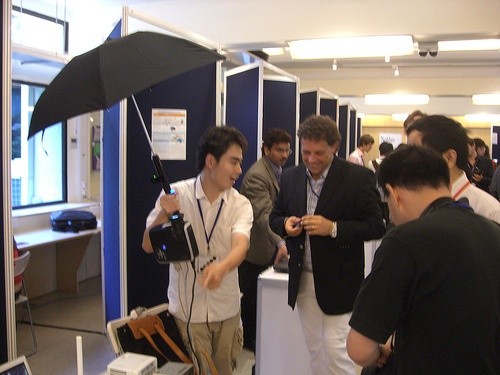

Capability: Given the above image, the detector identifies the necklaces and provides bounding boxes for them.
[453,182,470,199]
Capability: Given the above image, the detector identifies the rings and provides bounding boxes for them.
[312,224,315,229]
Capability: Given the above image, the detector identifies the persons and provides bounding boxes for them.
[369,142,393,224]
[268,115,386,375]
[466,137,500,203]
[403,111,426,127]
[142,126,254,375]
[13,236,22,300]
[346,146,500,375]
[240,127,292,375]
[349,134,375,166]
[407,115,500,224]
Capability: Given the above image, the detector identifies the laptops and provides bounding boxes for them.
[0,355,33,375]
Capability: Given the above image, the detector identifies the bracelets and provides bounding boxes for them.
[330,222,335,235]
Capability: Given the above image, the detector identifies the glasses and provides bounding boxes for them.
[272,148,293,155]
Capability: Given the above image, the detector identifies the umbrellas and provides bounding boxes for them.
[28,31,226,193]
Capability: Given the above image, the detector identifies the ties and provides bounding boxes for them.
[361,156,365,167]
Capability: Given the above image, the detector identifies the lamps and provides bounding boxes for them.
[266,32,500,121]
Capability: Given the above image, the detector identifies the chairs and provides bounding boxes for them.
[14,250,37,360]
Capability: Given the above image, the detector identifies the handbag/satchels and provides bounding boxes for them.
[50,210,97,233]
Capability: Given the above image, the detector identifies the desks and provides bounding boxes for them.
[15,219,100,297]
[255,268,310,375]
